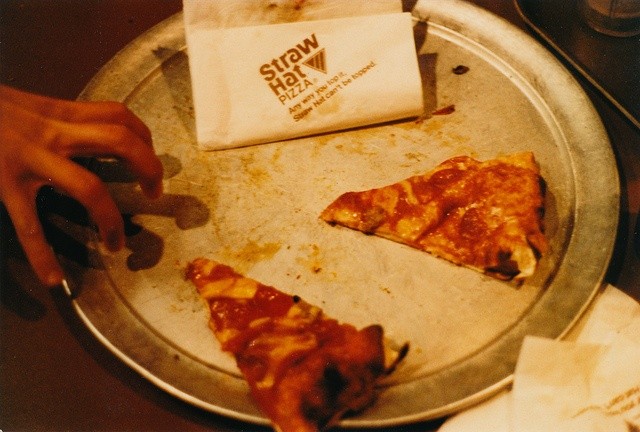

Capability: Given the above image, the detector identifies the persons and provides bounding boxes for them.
[1,84,163,288]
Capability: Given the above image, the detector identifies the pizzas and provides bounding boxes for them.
[320,149,543,288]
[188,256,409,432]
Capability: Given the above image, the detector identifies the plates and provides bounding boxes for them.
[57,3,625,428]
